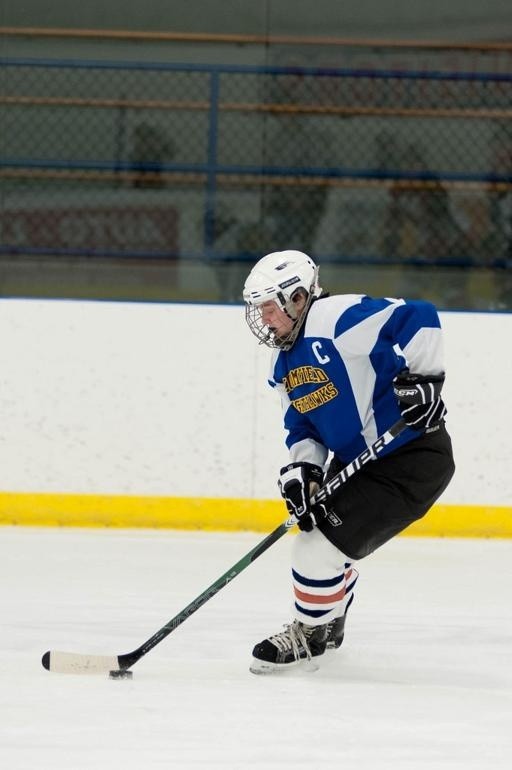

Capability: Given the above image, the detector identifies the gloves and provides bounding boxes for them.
[393,369,453,436]
[272,458,332,530]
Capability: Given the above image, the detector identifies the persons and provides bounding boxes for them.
[242,250,456,664]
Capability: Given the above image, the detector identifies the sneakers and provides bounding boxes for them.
[332,607,350,649]
[251,618,332,666]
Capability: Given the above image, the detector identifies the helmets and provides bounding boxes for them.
[236,248,321,354]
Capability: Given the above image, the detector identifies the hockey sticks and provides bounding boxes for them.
[42,421,409,675]
